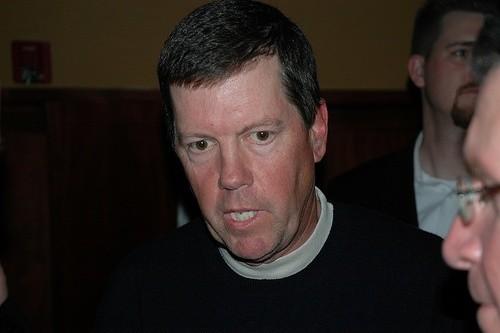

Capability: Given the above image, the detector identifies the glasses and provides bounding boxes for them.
[455,176,496,223]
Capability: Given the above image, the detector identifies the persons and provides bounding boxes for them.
[325,0,500,240]
[94,0,478,333]
[440,13,500,333]
